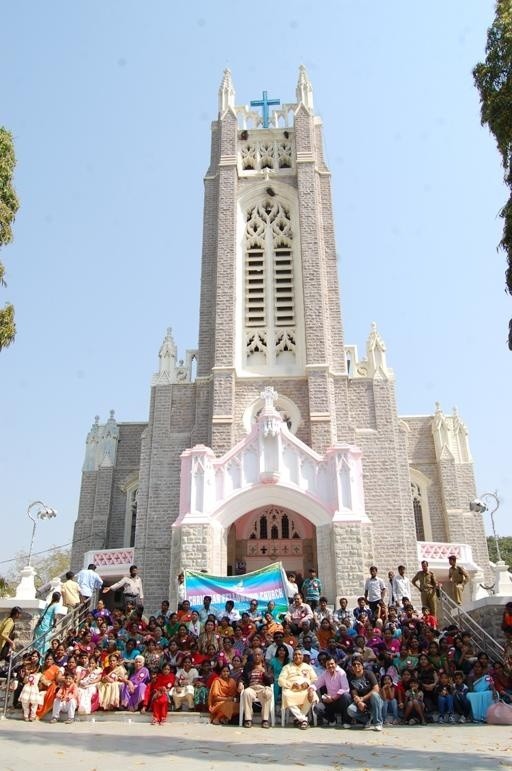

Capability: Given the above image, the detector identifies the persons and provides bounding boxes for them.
[364,567,386,608]
[447,556,469,605]
[177,574,185,605]
[1,607,22,679]
[283,569,300,603]
[103,565,144,608]
[33,592,62,655]
[301,568,322,609]
[412,561,436,615]
[18,595,512,733]
[62,572,84,610]
[73,564,104,603]
[392,566,410,607]
[387,571,397,606]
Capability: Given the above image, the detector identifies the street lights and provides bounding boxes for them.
[16,501,57,600]
[468,490,512,594]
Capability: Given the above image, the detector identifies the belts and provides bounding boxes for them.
[125,593,137,598]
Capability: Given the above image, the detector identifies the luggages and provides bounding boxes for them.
[467,691,498,721]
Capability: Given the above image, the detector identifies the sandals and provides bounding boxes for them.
[211,717,229,725]
[299,720,310,730]
[292,719,301,729]
[261,720,269,729]
[244,719,252,728]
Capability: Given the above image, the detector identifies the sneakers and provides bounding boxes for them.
[363,715,383,732]
[141,709,166,725]
[329,719,336,727]
[342,721,352,729]
[437,714,472,724]
[385,719,426,726]
[25,716,74,725]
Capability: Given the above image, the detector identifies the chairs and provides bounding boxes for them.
[239,684,275,726]
[281,687,317,727]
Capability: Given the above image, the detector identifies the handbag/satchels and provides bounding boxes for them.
[473,675,493,691]
[435,588,440,599]
[487,701,512,725]
